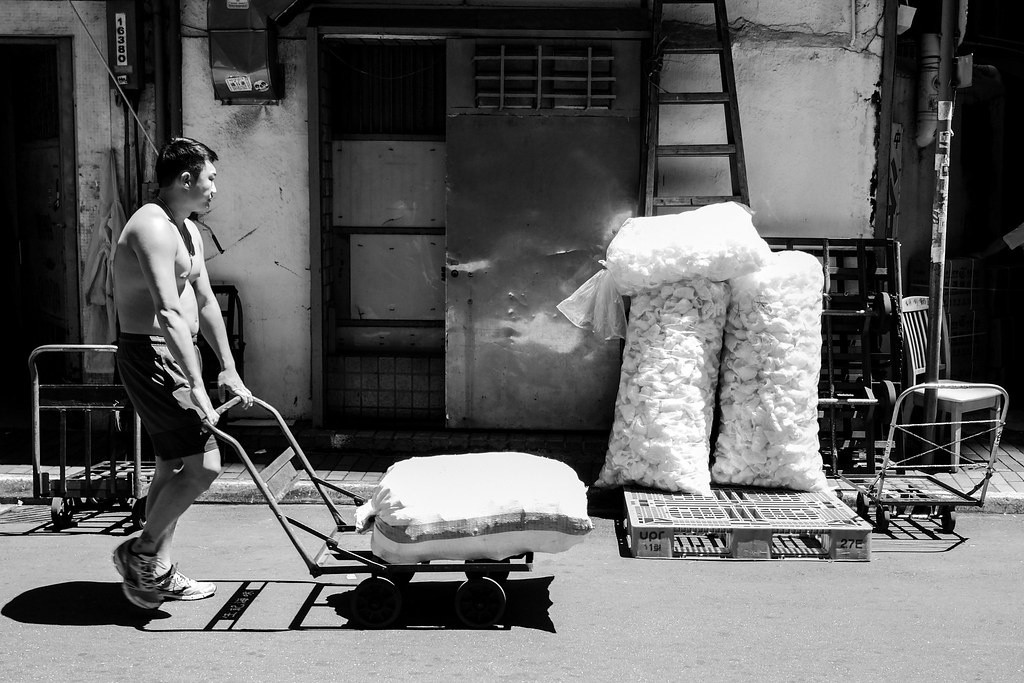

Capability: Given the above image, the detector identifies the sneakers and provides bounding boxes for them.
[155,562,218,603]
[111,536,167,610]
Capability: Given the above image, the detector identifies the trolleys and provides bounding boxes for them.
[839,381,1009,533]
[28,344,159,530]
[201,393,535,626]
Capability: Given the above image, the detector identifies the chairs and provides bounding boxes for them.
[899,294,1004,472]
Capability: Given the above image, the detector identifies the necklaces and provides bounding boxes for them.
[158,196,196,256]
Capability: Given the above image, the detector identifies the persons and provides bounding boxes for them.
[113,137,253,609]
